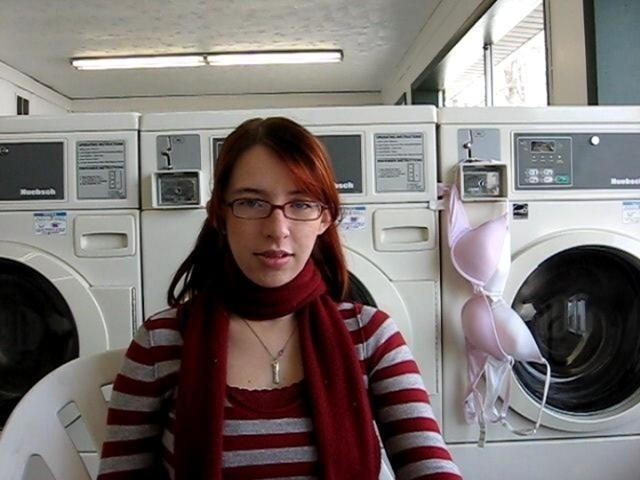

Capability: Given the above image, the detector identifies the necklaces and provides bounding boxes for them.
[235,314,300,385]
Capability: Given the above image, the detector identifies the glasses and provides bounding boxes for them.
[223,198,328,221]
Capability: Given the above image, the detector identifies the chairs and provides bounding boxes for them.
[1,351,129,479]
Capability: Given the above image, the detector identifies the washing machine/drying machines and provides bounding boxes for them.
[142,105,442,480]
[0,112,141,479]
[438,105,640,480]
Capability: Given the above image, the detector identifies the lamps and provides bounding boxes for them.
[69,50,346,72]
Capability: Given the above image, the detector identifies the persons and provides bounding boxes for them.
[95,118,463,480]
